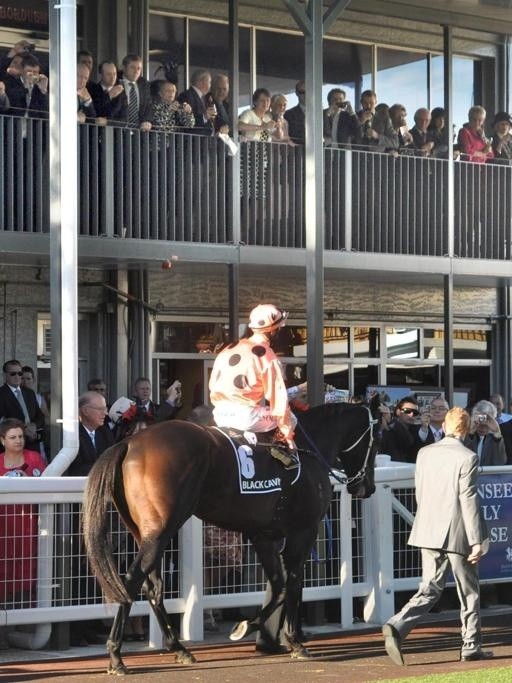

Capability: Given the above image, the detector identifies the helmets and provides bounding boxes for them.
[247,303,287,333]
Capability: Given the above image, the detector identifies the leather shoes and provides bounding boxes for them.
[460,652,494,662]
[382,623,405,667]
[122,633,145,642]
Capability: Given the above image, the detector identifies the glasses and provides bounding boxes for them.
[93,388,104,392]
[6,372,23,377]
[400,406,419,417]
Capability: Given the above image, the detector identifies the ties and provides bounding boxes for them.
[126,83,138,128]
[14,389,30,425]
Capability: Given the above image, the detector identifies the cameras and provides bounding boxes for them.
[476,415,487,423]
[336,101,347,109]
[176,387,181,393]
[31,74,39,82]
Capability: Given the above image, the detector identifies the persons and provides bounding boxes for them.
[1,38,512,259]
[204,292,300,466]
[381,406,490,669]
[4,356,507,640]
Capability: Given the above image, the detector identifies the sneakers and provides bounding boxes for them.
[70,623,110,646]
[271,440,298,468]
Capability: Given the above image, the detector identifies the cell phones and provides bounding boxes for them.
[426,404,431,418]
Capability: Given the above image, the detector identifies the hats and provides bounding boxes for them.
[491,112,512,126]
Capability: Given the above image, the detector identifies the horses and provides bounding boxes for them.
[79,393,383,676]
